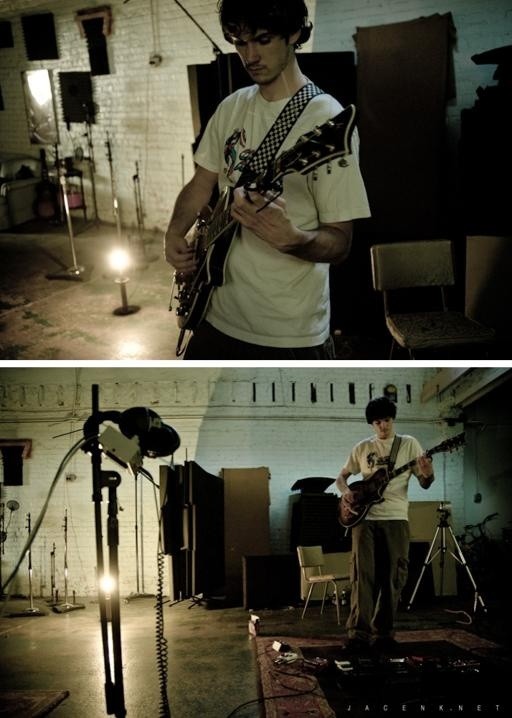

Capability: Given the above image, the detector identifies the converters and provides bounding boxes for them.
[272,640,291,652]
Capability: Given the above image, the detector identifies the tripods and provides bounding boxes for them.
[407,516,488,613]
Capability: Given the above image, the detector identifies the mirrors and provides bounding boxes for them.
[21,69,59,144]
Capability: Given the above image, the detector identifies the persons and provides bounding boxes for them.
[334,395,434,654]
[161,0,371,361]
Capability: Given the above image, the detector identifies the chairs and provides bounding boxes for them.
[296,545,350,625]
[370,239,495,360]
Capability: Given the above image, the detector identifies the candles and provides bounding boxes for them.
[106,248,132,281]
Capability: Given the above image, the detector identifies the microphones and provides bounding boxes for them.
[168,453,176,471]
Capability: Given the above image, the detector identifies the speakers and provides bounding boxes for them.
[242,554,300,610]
[289,493,352,555]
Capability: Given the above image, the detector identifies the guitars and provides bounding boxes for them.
[175,103,358,329]
[32,148,59,219]
[337,433,464,528]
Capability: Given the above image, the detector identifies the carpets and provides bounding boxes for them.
[252,626,512,718]
[0,690,69,718]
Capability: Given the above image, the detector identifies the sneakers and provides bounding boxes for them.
[342,636,392,648]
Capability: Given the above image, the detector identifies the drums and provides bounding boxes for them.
[66,192,82,208]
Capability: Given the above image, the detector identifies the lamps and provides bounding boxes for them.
[149,54,162,67]
